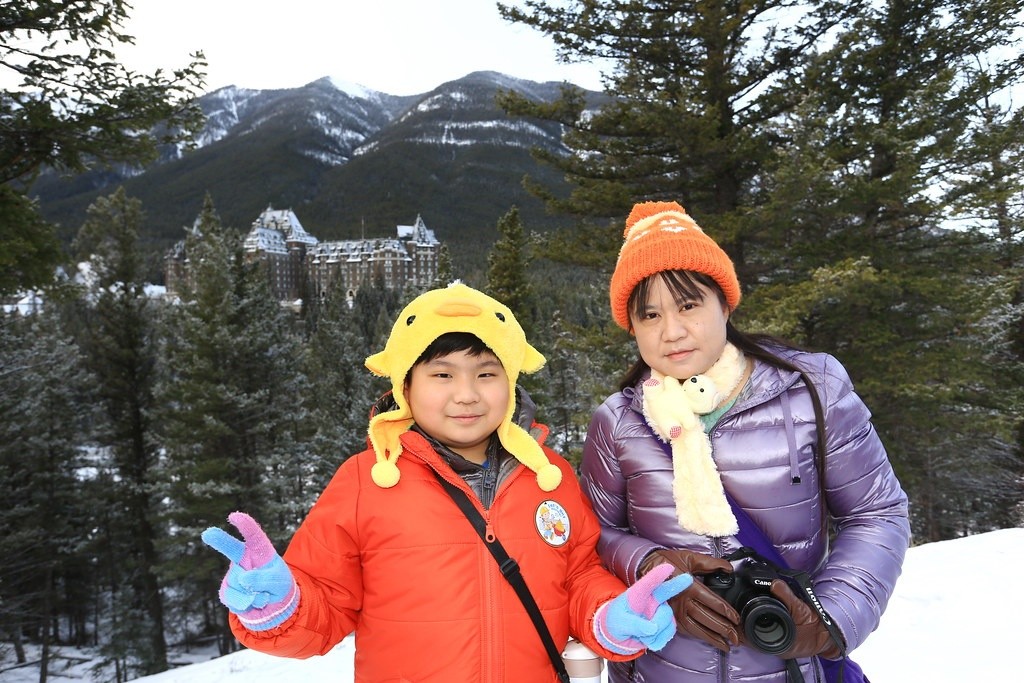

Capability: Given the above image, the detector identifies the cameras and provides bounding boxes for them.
[701,560,797,657]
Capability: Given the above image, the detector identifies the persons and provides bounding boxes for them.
[578,202,910,683]
[201,280,693,683]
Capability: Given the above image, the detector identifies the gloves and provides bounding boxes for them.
[639,548,740,654]
[735,579,844,661]
[200,511,299,632]
[592,562,693,656]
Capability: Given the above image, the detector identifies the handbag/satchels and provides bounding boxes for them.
[822,656,870,683]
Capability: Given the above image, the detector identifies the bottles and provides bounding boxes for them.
[561,640,604,683]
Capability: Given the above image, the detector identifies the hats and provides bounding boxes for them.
[365,280,562,492]
[610,201,741,336]
